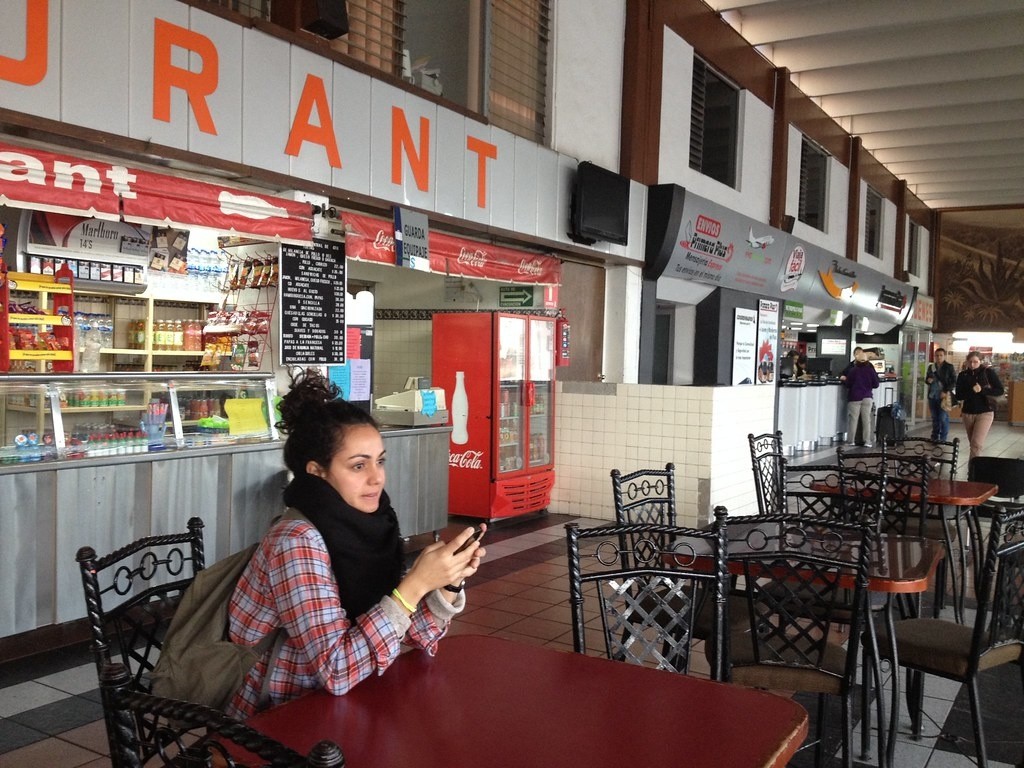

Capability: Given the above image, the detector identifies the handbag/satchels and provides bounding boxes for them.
[981,366,1002,412]
[940,387,963,413]
[144,505,332,732]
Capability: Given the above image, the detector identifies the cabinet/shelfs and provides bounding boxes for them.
[0,263,73,374]
[0,290,236,445]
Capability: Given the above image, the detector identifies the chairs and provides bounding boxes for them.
[75,515,347,768]
[563,429,1024,768]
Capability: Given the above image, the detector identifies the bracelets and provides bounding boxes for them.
[393,589,417,612]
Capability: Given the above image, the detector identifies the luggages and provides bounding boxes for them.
[873,387,905,447]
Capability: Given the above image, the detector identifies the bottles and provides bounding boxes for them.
[55,310,215,372]
[497,380,548,472]
[450,371,470,445]
[167,394,222,420]
[8,390,149,463]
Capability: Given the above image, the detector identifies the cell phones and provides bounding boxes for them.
[454,527,482,554]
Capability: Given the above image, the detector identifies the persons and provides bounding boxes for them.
[925,348,956,441]
[787,350,808,377]
[224,368,486,722]
[840,347,879,448]
[956,351,1004,457]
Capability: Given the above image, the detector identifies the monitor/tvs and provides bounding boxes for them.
[571,160,630,248]
[779,357,794,380]
[806,358,831,380]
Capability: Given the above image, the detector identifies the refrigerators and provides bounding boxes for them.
[429,312,557,526]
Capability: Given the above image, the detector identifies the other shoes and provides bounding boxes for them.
[863,443,873,448]
[844,442,856,446]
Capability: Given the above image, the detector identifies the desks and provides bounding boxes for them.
[809,474,999,626]
[658,520,945,768]
[208,635,809,768]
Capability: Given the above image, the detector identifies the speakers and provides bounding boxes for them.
[302,0,348,41]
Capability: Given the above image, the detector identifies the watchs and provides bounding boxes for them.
[444,579,465,593]
